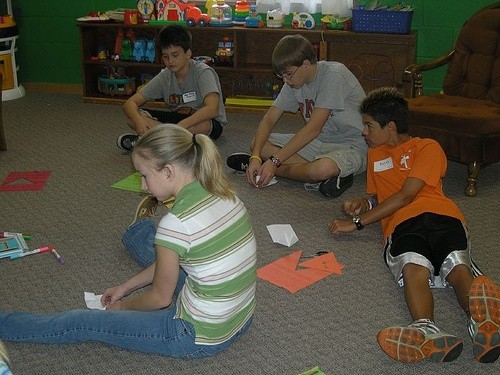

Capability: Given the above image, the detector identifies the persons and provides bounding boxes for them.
[327,87,500,363]
[0,123,257,358]
[116,24,228,151]
[226,34,370,197]
[0,341,13,375]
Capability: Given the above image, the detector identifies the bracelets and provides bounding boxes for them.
[249,155,262,164]
[363,198,376,212]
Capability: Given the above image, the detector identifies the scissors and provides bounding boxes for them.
[301,251,329,257]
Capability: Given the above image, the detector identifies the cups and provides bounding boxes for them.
[124,10,137,25]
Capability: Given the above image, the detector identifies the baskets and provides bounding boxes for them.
[352,9,414,34]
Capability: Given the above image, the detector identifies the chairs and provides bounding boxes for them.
[402,2,500,195]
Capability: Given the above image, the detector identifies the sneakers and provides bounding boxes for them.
[227,152,251,174]
[376,318,463,365]
[467,275,500,363]
[117,132,138,151]
[128,195,159,227]
[319,172,354,198]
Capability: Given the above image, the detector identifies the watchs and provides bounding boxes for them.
[269,155,282,167]
[352,215,364,230]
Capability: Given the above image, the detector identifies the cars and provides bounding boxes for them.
[186,7,210,27]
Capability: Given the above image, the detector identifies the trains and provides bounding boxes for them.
[214,35,233,66]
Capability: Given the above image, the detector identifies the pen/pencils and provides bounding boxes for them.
[0,232,32,240]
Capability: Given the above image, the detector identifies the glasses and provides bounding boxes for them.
[275,66,300,80]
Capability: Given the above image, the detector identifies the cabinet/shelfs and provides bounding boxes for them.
[77,20,418,116]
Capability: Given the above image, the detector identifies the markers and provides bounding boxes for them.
[10,247,49,259]
[50,246,65,264]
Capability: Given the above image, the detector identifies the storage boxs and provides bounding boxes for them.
[98,76,135,94]
